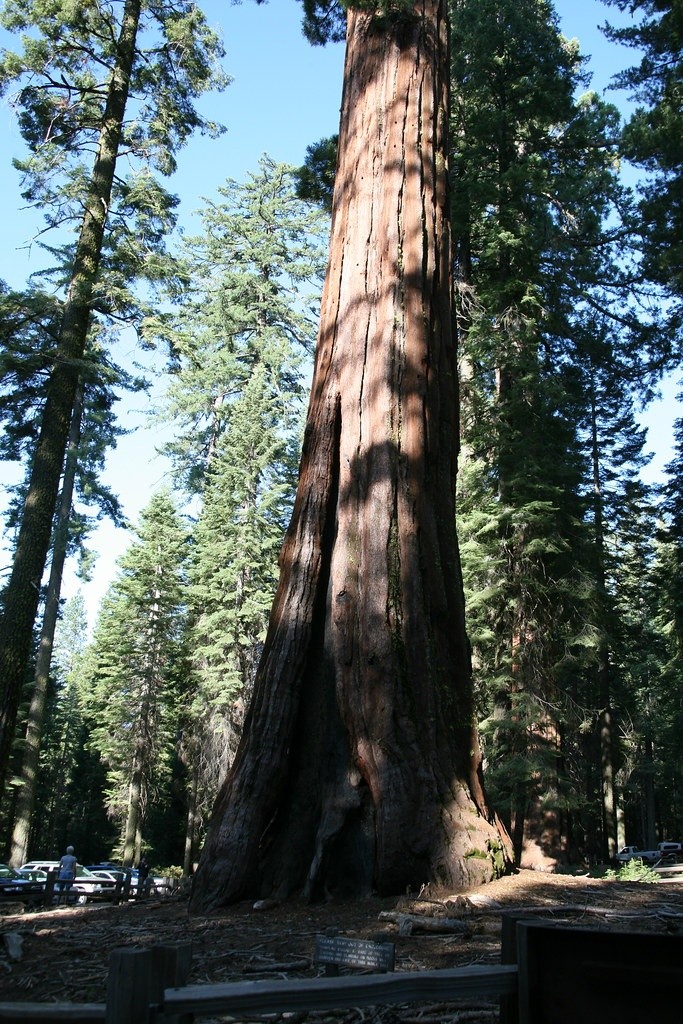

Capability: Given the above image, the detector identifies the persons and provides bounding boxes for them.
[135,857,151,881]
[57,846,76,905]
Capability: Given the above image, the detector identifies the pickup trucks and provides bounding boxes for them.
[615,846,661,867]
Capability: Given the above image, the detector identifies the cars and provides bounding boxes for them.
[85,865,153,883]
[657,842,683,863]
[20,861,117,904]
[0,868,79,906]
[0,863,45,906]
[82,870,157,899]
